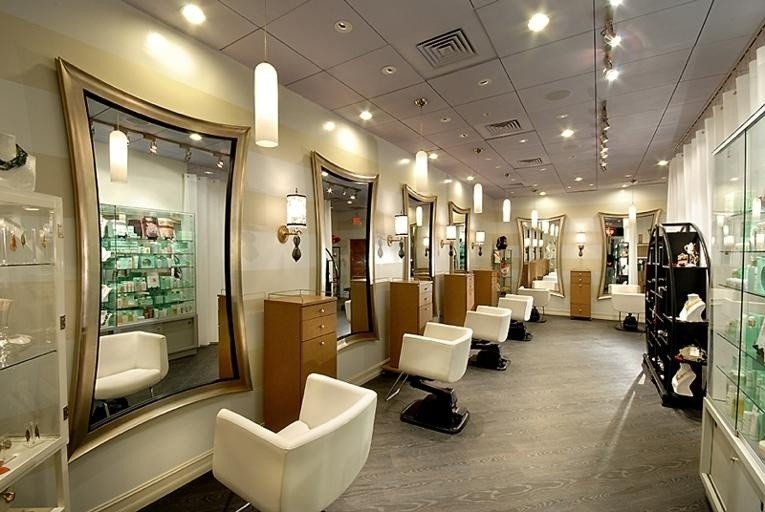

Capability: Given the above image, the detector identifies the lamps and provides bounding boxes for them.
[524,239,543,248]
[629,204,636,221]
[599,0,620,175]
[276,188,486,262]
[538,221,559,237]
[575,231,586,257]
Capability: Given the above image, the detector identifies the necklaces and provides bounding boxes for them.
[676,370,688,380]
[685,298,702,311]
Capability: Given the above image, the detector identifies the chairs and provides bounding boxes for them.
[612,293,646,332]
[532,269,557,290]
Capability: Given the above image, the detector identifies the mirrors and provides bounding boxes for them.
[516,213,565,299]
[597,209,662,300]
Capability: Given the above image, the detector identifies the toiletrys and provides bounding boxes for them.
[723,191,765,462]
[103,239,193,327]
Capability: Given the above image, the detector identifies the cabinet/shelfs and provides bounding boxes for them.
[264,269,497,432]
[699,104,765,511]
[570,270,592,320]
[0,189,71,512]
[493,249,512,298]
[642,221,710,411]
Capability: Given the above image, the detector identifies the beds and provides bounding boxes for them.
[608,284,640,294]
